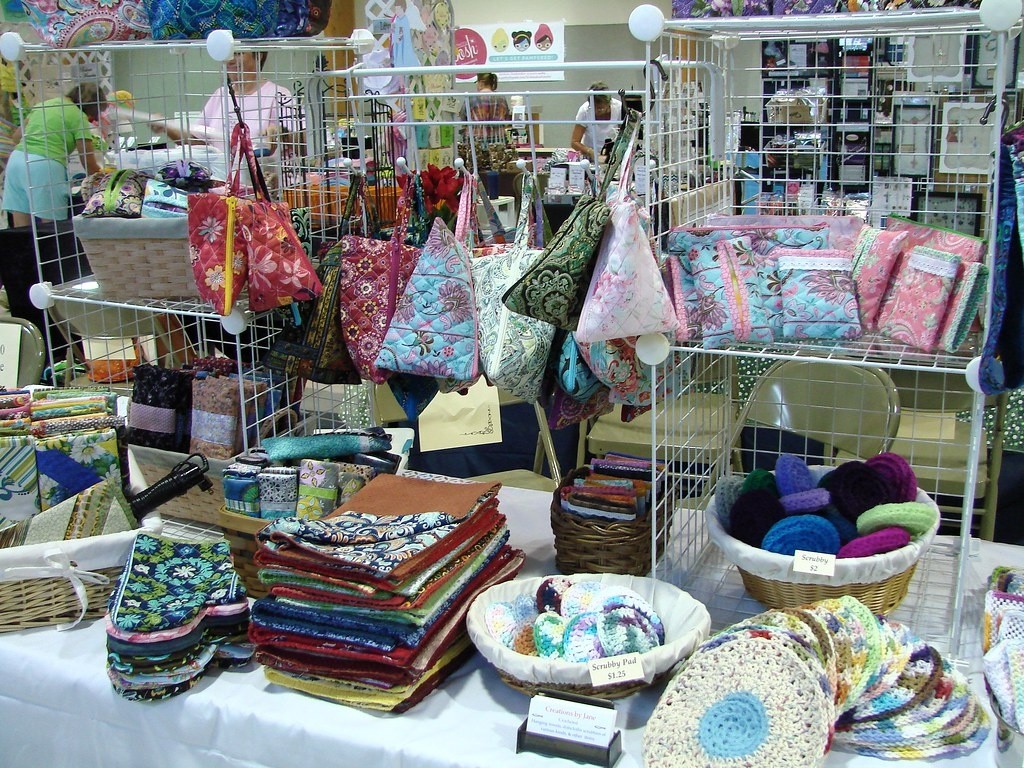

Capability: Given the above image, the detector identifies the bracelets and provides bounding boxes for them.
[587,148,593,155]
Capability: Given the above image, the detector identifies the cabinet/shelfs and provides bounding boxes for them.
[631,1,1022,662]
[0,30,375,457]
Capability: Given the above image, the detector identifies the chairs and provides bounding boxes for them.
[27,280,182,386]
[577,355,746,496]
[375,375,562,493]
[703,354,901,496]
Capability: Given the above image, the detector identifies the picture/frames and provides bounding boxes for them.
[971,32,1020,89]
[511,106,544,148]
[870,169,916,219]
[938,102,994,174]
[891,104,934,177]
[907,29,967,82]
[911,191,983,237]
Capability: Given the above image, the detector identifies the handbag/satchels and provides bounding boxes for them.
[82,159,217,217]
[186,107,680,428]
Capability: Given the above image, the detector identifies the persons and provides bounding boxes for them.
[571,82,621,160]
[2,82,108,227]
[146,51,306,195]
[461,74,510,150]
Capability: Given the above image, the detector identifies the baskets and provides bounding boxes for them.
[551,465,670,578]
[72,197,198,298]
[466,573,711,699]
[0,448,162,634]
[705,465,941,622]
[127,394,317,528]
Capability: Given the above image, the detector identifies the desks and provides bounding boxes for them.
[0,443,1024,768]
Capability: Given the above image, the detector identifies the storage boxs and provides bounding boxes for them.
[130,442,249,526]
[765,98,828,123]
